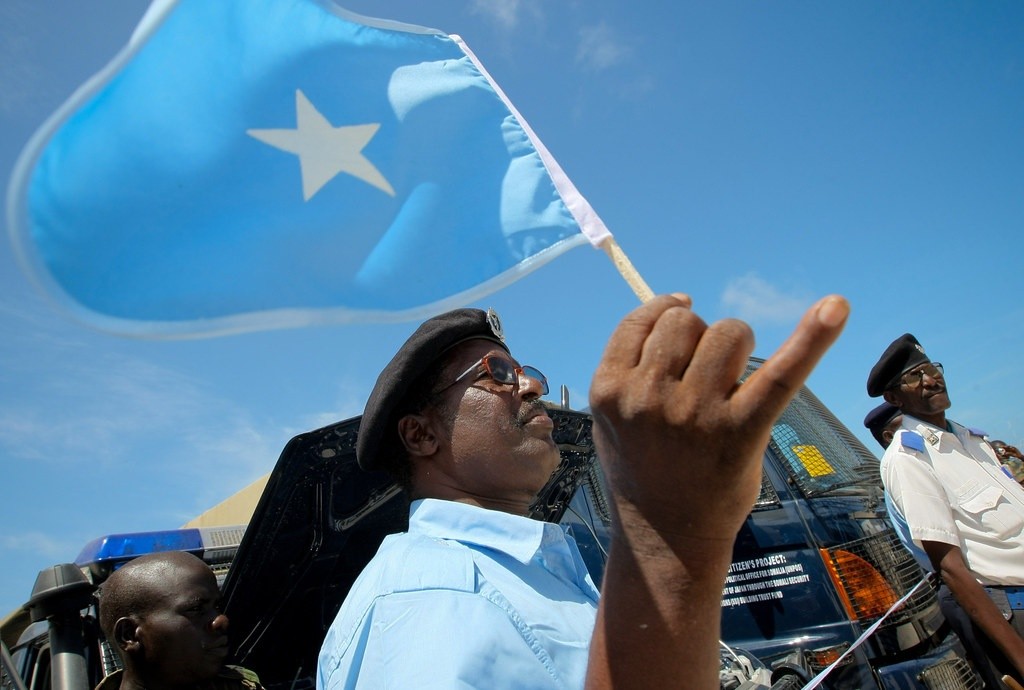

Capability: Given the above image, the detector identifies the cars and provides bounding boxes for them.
[0,398,594,689]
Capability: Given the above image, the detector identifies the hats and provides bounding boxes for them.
[355,306,511,471]
[864,401,902,432]
[867,333,930,397]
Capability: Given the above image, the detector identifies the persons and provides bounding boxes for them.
[865,334,1024,690]
[94,551,266,690]
[315,292,849,690]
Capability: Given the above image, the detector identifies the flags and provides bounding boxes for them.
[6,1,613,339]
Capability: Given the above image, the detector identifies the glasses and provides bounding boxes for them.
[884,362,944,391]
[420,350,550,409]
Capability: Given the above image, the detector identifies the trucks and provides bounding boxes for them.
[559,356,986,690]
